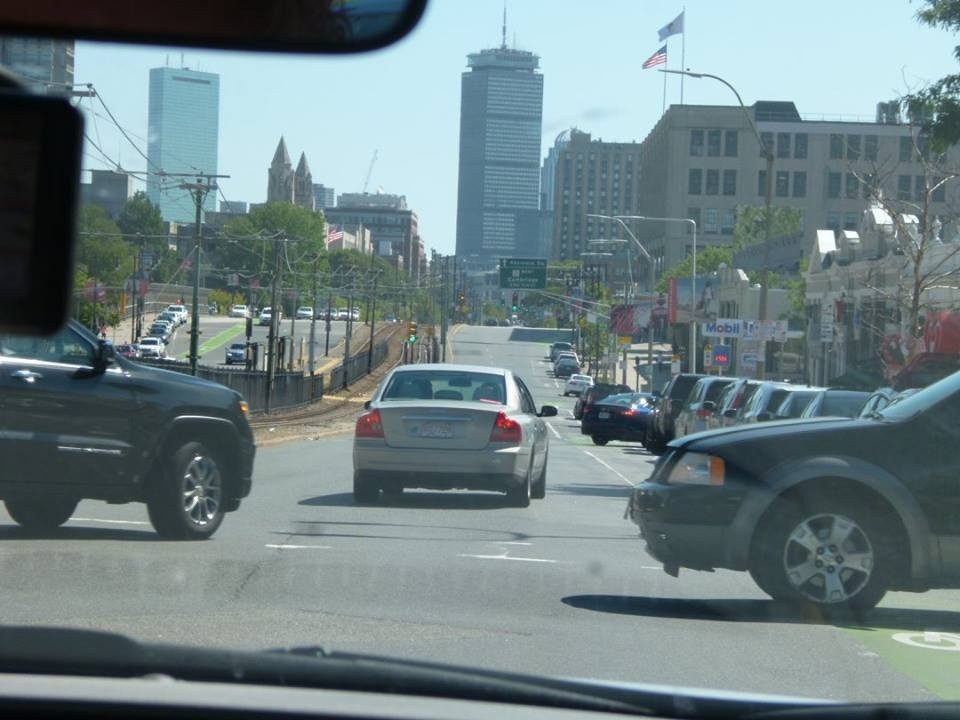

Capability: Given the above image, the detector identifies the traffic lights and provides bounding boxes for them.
[460,293,464,304]
[407,322,417,343]
[512,292,518,313]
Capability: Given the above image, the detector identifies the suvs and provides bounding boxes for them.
[644,369,960,621]
[0,315,256,542]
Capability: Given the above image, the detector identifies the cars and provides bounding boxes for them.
[296,306,359,321]
[565,374,594,396]
[231,305,248,317]
[485,318,510,327]
[225,343,249,364]
[573,383,657,446]
[353,365,558,507]
[115,304,187,357]
[549,341,580,377]
[259,307,282,324]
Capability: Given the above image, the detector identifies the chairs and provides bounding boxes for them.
[434,389,463,400]
[473,382,502,401]
[399,383,423,397]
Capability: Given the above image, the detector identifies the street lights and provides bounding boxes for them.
[612,214,696,374]
[658,67,774,380]
[585,214,657,395]
